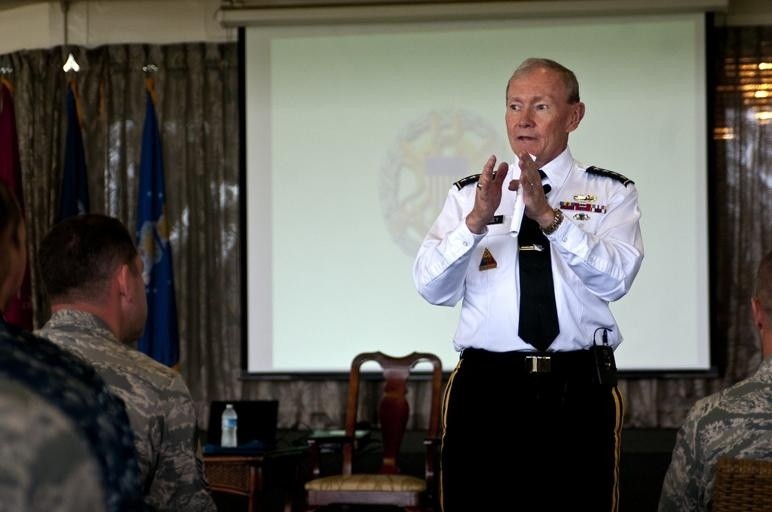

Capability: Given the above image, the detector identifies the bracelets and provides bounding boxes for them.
[544,208,563,235]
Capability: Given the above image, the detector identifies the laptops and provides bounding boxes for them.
[201,400,279,457]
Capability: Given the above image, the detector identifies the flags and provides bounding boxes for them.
[2,77,178,370]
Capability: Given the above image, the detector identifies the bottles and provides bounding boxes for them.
[221,404,238,449]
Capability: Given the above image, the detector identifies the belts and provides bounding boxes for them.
[462,355,594,374]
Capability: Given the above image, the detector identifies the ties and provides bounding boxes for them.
[518,169,560,352]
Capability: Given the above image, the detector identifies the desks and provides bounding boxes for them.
[201,428,428,512]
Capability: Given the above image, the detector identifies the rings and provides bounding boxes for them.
[477,183,482,187]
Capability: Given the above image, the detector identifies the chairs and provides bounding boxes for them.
[712,456,772,512]
[304,352,441,512]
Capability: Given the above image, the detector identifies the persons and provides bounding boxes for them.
[412,59,644,512]
[659,252,772,512]
[1,178,216,512]
